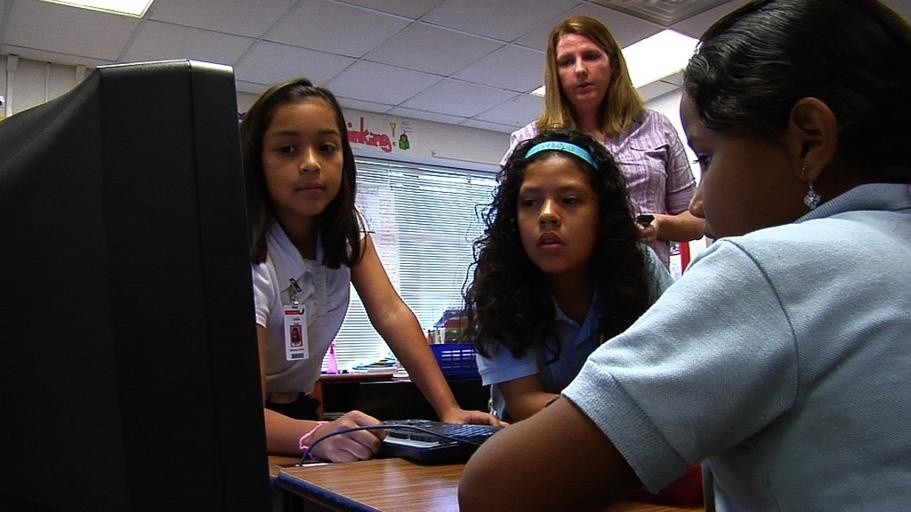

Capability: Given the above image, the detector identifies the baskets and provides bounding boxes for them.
[428,344,481,380]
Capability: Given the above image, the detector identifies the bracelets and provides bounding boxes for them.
[298,419,330,459]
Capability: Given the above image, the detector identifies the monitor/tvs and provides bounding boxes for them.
[1,58,279,512]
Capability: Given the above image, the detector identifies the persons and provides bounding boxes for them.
[291,327,302,347]
[457,0,911,511]
[463,126,678,423]
[493,16,707,273]
[239,75,511,463]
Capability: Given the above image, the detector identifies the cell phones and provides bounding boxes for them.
[637,215,655,227]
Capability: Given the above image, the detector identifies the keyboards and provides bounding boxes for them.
[379,418,504,465]
